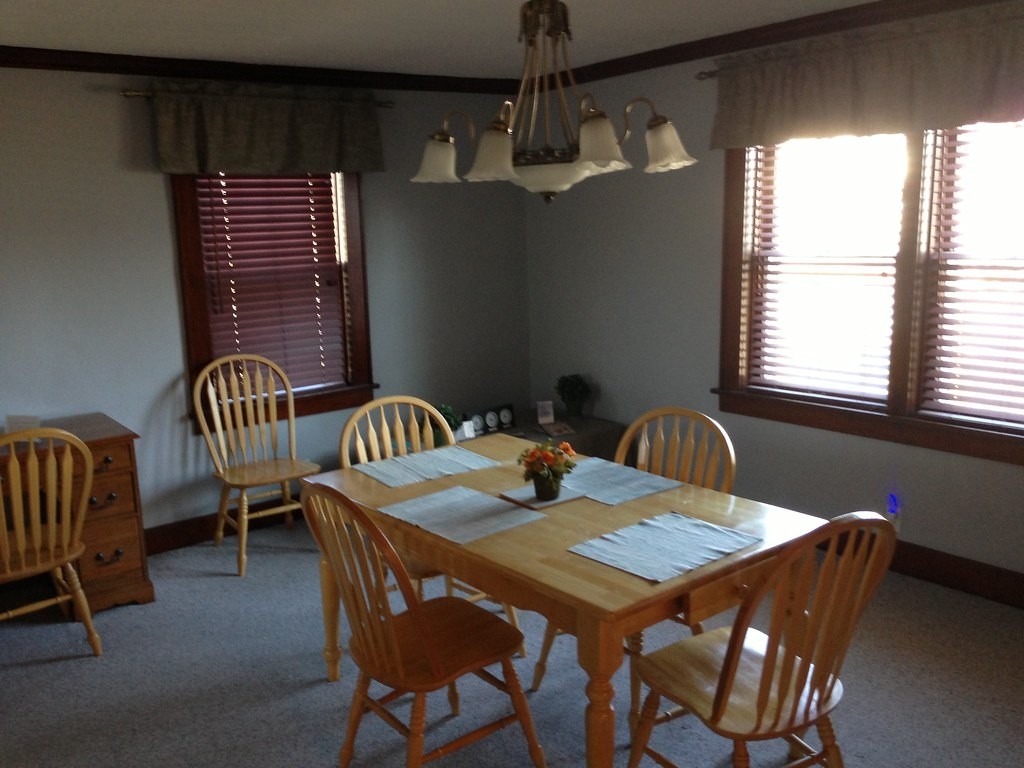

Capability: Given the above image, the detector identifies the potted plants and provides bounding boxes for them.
[554,372,589,418]
[416,403,463,449]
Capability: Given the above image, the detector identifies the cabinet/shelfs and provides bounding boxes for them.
[0,409,159,616]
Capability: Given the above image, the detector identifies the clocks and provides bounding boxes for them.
[467,403,517,433]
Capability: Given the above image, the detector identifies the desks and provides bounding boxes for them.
[381,413,627,462]
[301,433,830,768]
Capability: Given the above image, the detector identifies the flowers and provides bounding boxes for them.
[517,438,576,488]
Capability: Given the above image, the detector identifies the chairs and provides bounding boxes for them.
[301,483,548,768]
[0,427,105,657]
[337,396,525,659]
[531,408,736,747]
[193,353,324,576]
[621,512,896,768]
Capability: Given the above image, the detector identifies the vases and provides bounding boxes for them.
[534,472,561,499]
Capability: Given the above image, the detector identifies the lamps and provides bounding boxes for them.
[409,0,697,203]
